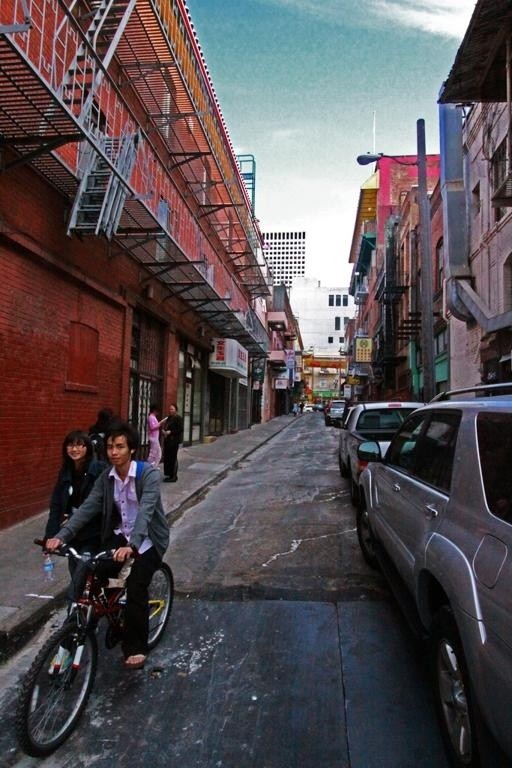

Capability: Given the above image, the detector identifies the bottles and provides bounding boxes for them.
[42,550,55,583]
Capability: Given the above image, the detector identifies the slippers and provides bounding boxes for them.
[125,652,149,669]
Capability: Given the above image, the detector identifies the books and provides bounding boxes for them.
[158,416,169,424]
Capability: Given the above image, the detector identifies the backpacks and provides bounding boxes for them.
[88,433,106,460]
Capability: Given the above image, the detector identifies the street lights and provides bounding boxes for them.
[356,149,437,401]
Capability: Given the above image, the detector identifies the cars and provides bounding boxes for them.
[303,399,347,427]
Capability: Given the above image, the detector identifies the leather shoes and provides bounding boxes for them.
[163,476,177,482]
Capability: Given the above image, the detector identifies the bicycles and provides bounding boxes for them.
[18,537,173,757]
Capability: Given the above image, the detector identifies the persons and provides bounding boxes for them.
[145,402,169,471]
[43,418,171,672]
[161,402,184,483]
[292,401,298,416]
[300,402,304,415]
[41,428,110,686]
[87,406,117,466]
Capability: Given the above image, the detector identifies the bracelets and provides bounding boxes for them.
[126,542,139,558]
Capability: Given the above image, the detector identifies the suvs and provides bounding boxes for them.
[355,379,511,766]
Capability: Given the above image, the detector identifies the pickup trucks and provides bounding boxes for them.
[339,401,431,508]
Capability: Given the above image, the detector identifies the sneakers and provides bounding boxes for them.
[48,654,73,675]
